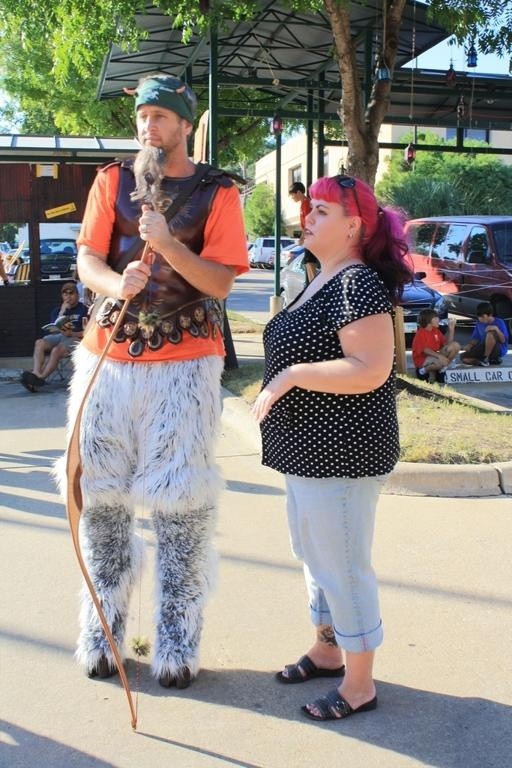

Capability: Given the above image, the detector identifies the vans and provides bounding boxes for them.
[401,212,512,324]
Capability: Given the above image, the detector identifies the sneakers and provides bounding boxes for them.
[436,370,446,383]
[478,355,489,367]
[490,356,502,364]
[415,367,429,381]
[20,371,46,392]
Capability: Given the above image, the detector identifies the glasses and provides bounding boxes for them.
[334,173,363,218]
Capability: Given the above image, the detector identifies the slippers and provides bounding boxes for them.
[300,687,377,721]
[276,653,346,683]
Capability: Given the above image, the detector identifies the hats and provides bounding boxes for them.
[61,283,78,293]
[123,74,198,122]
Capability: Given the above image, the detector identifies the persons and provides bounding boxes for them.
[287,181,319,285]
[249,171,416,726]
[0,251,14,290]
[457,299,509,368]
[48,72,255,694]
[18,281,91,396]
[410,308,462,385]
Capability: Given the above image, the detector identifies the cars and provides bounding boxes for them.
[387,269,453,350]
[245,236,308,270]
[0,233,78,280]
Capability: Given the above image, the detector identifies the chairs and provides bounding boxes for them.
[48,308,84,383]
